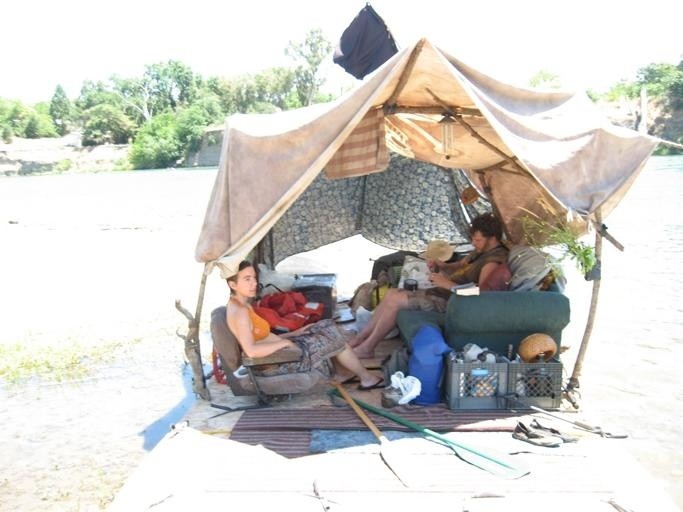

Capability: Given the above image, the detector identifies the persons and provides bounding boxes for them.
[346,212,510,360]
[227,259,386,391]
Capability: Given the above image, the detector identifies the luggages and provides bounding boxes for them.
[371,284,391,308]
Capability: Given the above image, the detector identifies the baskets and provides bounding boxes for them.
[444,352,507,411]
[508,356,562,409]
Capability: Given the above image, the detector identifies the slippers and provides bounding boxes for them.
[359,376,384,390]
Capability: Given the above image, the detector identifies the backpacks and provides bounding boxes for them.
[348,280,376,317]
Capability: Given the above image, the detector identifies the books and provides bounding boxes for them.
[449,281,480,297]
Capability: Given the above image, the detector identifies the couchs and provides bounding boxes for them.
[387,246,570,359]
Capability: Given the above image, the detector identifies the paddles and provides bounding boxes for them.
[324,375,443,489]
[328,384,531,479]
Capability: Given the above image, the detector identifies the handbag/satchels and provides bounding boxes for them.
[381,342,409,384]
[403,325,451,407]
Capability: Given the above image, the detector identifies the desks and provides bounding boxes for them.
[292,272,338,320]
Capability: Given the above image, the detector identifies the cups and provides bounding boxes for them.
[403,278,418,291]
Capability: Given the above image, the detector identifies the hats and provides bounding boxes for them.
[422,240,454,263]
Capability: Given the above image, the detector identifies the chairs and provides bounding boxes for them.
[210,306,319,406]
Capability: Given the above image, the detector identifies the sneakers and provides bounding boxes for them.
[529,419,577,442]
[381,386,403,407]
[512,422,564,446]
